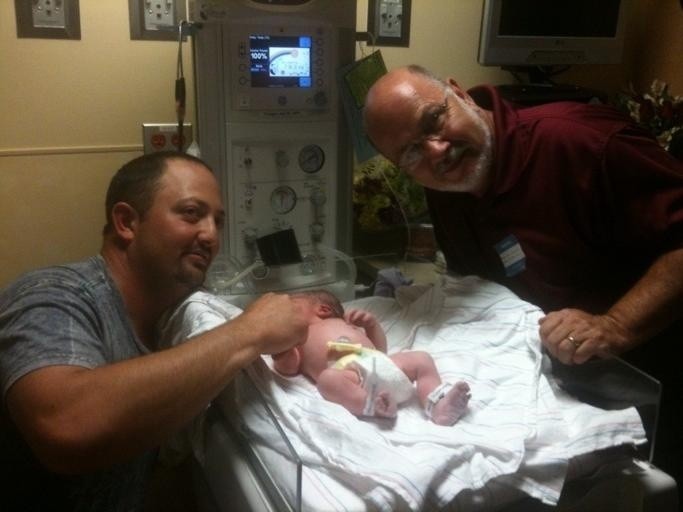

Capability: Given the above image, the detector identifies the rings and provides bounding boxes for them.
[566,336,579,348]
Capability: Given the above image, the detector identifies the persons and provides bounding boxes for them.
[270,288,473,427]
[360,63,683,511]
[0,151,312,512]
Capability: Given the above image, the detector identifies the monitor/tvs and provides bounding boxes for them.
[476,0,623,66]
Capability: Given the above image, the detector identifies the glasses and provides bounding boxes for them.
[396,89,456,183]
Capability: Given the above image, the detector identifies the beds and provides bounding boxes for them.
[180,282,664,511]
